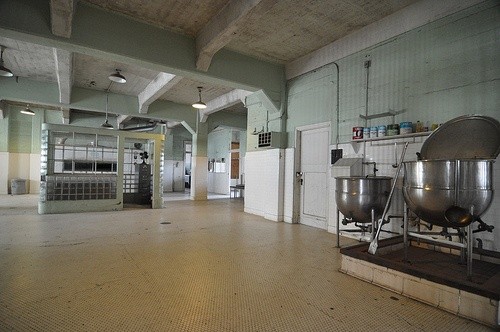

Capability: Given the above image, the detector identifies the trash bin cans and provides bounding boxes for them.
[11,179,27,195]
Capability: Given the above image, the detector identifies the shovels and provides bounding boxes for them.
[368,141,410,255]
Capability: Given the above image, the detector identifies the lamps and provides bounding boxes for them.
[191,87,207,108]
[0,45,14,77]
[109,69,127,83]
[19,103,36,115]
[100,93,113,127]
